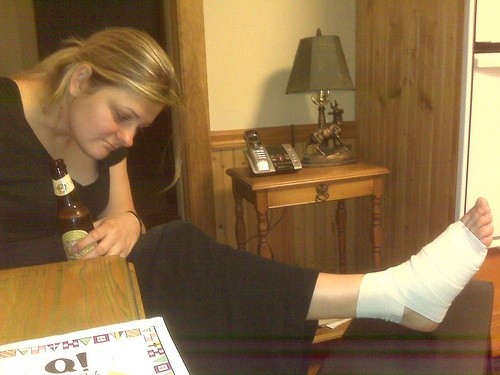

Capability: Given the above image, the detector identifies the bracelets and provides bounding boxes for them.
[126,210,143,237]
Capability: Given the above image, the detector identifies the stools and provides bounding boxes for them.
[315,279,494,375]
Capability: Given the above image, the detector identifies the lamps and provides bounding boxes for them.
[286,28,358,167]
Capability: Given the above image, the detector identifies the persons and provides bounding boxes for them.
[0,27,495,375]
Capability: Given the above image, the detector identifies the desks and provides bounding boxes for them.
[225,161,391,273]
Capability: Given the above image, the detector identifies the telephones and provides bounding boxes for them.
[243,124,302,177]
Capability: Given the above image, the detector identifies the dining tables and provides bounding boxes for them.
[0,255,147,345]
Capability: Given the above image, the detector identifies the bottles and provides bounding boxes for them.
[49,158,104,261]
